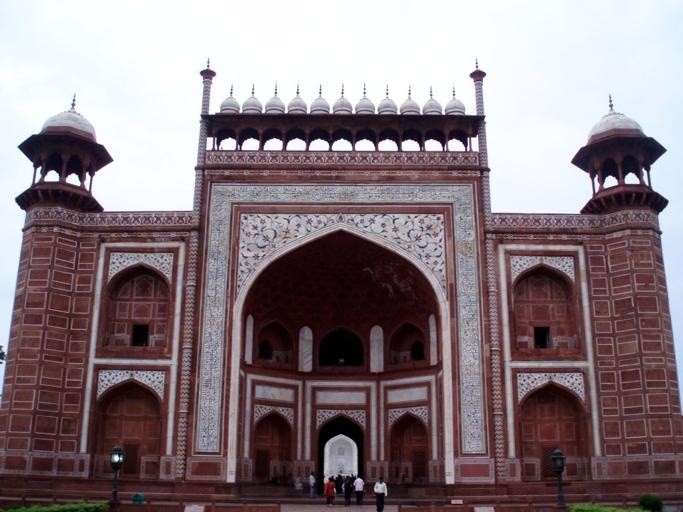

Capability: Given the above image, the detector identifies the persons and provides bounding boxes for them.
[324,473,365,507]
[308,471,317,496]
[373,476,388,512]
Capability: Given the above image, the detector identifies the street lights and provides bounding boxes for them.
[107,442,126,512]
[551,447,566,505]
